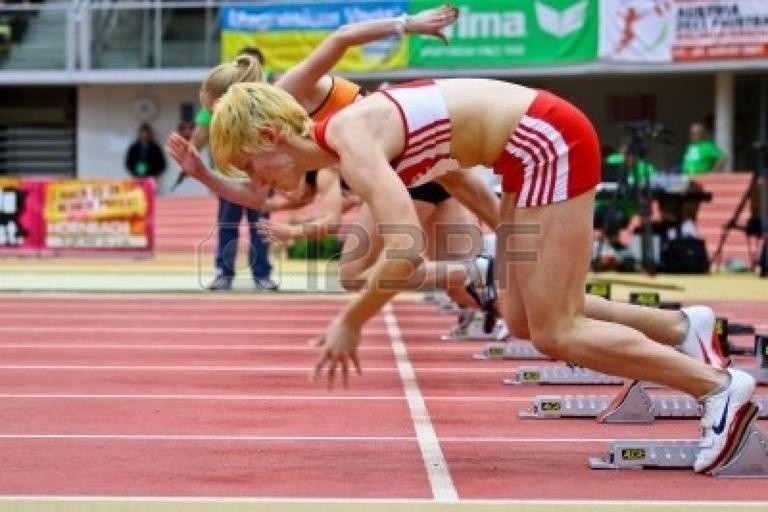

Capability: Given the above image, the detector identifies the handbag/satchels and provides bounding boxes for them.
[662,235,708,273]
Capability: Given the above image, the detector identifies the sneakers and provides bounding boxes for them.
[209,276,232,290]
[675,305,723,370]
[458,306,474,330]
[254,277,278,290]
[463,255,498,333]
[695,368,756,472]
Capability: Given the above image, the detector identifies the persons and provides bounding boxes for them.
[164,5,757,472]
[124,124,167,182]
